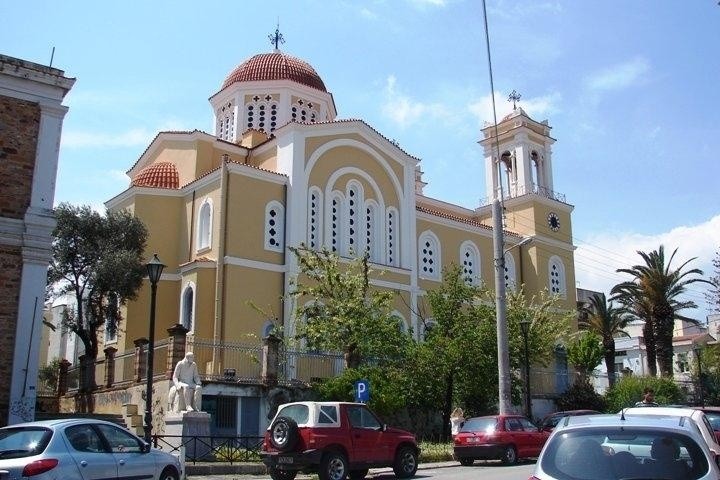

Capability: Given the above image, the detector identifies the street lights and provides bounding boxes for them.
[518,315,534,425]
[141,253,169,448]
[692,343,704,407]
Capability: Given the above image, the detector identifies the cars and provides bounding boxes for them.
[452,405,720,479]
[0,417,187,480]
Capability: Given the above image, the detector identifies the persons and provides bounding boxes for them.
[172,350,203,413]
[449,406,465,463]
[633,387,659,408]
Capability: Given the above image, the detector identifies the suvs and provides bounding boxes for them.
[258,400,423,480]
